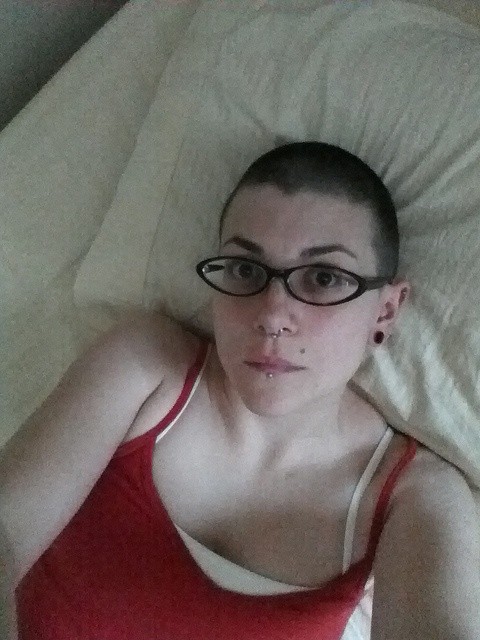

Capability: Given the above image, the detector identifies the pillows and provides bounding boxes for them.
[70,1,480,489]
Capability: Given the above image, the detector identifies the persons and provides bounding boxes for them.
[0,143,477,640]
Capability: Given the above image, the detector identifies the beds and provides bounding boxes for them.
[1,1,480,640]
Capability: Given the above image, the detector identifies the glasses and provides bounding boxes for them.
[194,254,397,308]
